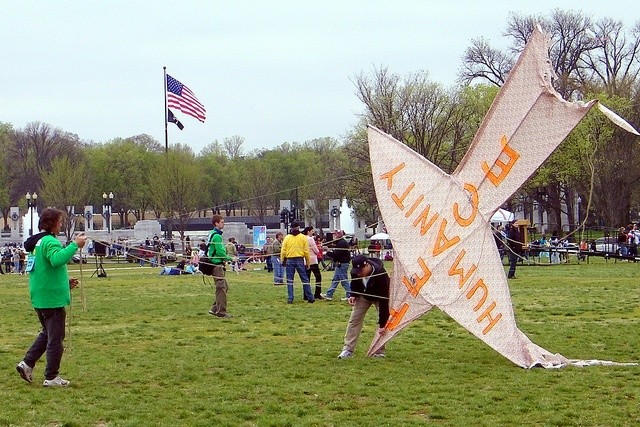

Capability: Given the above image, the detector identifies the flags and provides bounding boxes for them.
[168,107,187,130]
[167,75,209,123]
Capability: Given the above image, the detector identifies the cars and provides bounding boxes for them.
[127,246,168,264]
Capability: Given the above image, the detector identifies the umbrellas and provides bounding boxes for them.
[369,231,390,241]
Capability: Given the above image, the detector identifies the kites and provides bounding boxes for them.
[366,38,616,368]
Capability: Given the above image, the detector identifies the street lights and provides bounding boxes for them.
[102,191,114,232]
[25,191,38,235]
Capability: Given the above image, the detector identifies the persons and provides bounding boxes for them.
[628,224,635,263]
[18,211,71,390]
[376,241,381,258]
[263,236,273,272]
[384,251,393,261]
[538,231,596,262]
[303,225,323,299]
[0,240,26,274]
[315,235,328,270]
[508,218,522,279]
[617,226,629,256]
[321,229,351,301]
[273,232,284,284]
[368,242,375,254]
[280,221,315,304]
[494,225,503,257]
[86,233,207,275]
[338,256,390,359]
[210,214,233,316]
[225,238,236,275]
[633,223,640,262]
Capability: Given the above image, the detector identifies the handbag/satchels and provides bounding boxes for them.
[199,232,219,275]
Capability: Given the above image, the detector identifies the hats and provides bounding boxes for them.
[275,232,283,235]
[290,220,300,227]
[350,255,367,273]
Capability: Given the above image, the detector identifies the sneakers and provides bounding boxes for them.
[341,298,349,301]
[338,350,352,358]
[320,293,331,301]
[287,300,292,304]
[43,376,71,388]
[313,294,324,299]
[16,361,33,383]
[374,353,385,357]
[308,299,316,303]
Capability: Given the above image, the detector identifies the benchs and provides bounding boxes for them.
[367,248,380,260]
